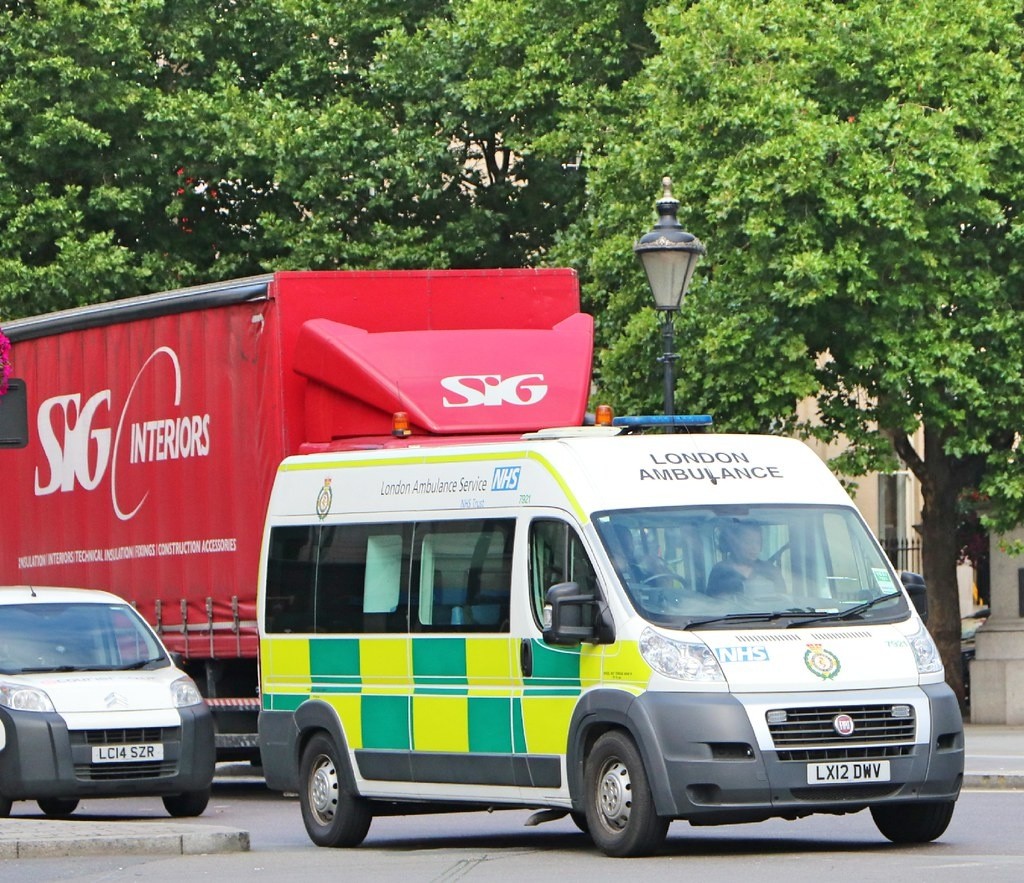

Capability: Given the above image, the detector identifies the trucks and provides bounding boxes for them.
[1,267,594,755]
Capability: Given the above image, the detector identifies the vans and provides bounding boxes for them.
[0,586,217,818]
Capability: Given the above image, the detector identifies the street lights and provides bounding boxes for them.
[635,176,707,431]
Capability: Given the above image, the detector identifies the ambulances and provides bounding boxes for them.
[256,406,965,857]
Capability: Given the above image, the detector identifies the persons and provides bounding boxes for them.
[708,524,789,600]
[593,524,675,642]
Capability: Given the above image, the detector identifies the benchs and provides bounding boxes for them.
[433,587,506,624]
